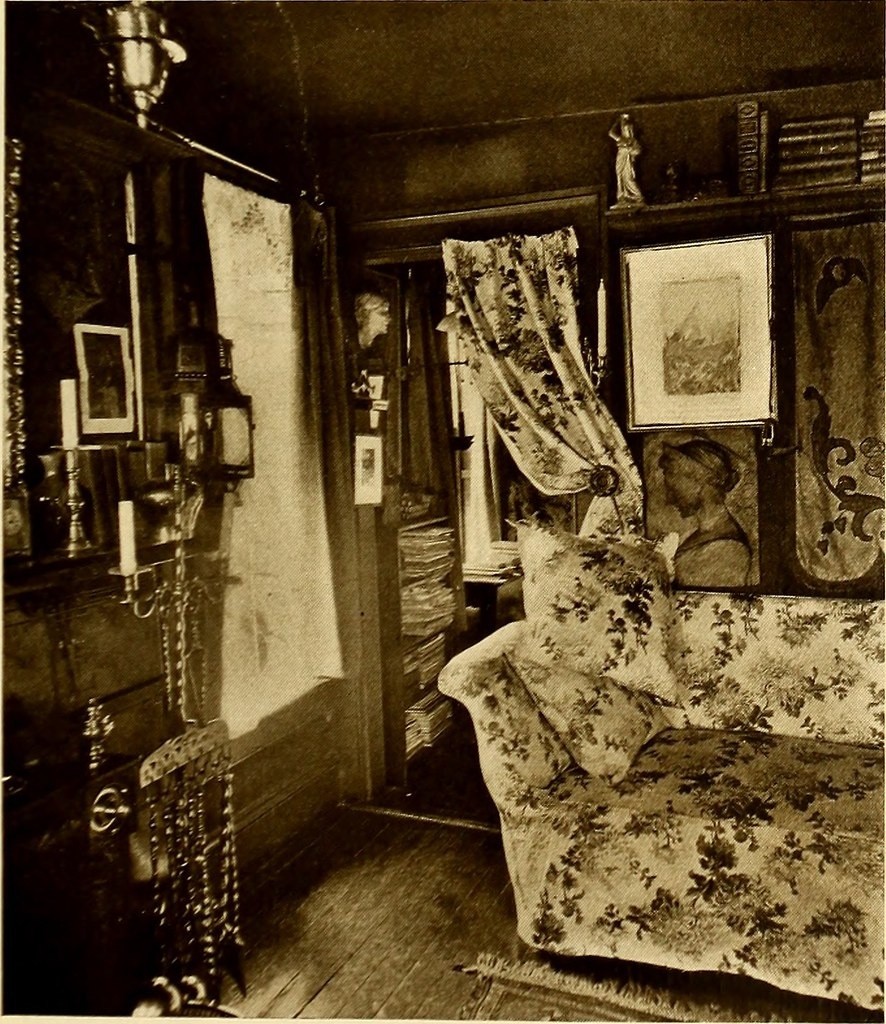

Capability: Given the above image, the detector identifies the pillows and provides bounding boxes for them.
[504,634,675,786]
[517,519,682,704]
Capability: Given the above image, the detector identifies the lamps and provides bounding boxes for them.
[80,3,189,129]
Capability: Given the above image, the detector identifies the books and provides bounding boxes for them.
[402,528,456,761]
[735,100,885,196]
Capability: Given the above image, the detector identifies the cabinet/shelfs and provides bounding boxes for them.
[376,514,464,787]
[4,751,141,1014]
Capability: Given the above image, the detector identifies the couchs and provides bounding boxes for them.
[437,590,885,1015]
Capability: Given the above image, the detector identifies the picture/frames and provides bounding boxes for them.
[352,268,395,433]
[353,434,384,508]
[620,231,778,432]
[73,322,135,435]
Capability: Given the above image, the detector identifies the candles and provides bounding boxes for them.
[118,499,137,574]
[59,378,79,452]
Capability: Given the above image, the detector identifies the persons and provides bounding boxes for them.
[607,114,647,204]
[355,292,391,349]
